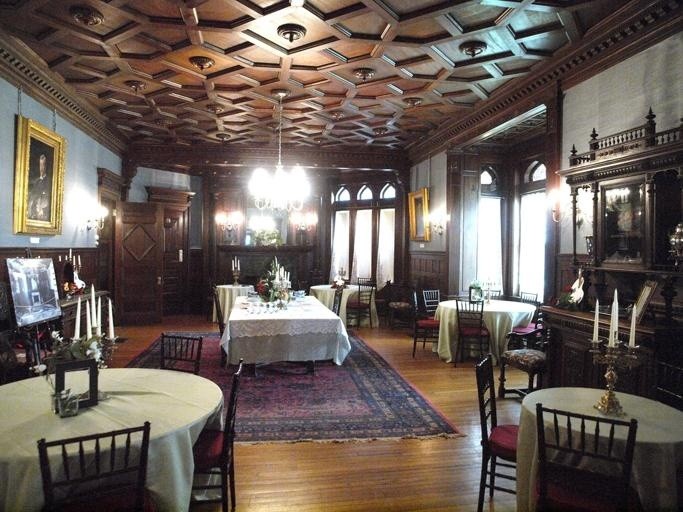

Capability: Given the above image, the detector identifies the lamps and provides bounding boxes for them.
[427,206,452,235]
[247,86,313,214]
[214,207,321,234]
[80,201,109,232]
[547,188,578,223]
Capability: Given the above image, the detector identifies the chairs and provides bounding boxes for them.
[347,276,376,331]
[189,357,244,512]
[411,286,543,363]
[533,401,639,511]
[329,286,345,316]
[159,331,204,376]
[209,285,229,366]
[35,420,154,511]
[472,351,526,511]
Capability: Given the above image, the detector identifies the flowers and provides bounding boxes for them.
[28,330,113,377]
[256,274,276,295]
[548,283,577,308]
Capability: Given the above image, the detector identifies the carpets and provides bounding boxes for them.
[125,330,469,448]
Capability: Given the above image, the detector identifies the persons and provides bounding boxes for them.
[27,152,49,218]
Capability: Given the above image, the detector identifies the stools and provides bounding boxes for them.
[498,346,553,404]
[390,298,414,330]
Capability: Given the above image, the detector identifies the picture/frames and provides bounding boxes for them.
[407,186,433,244]
[11,110,68,239]
[630,277,657,324]
[55,361,100,411]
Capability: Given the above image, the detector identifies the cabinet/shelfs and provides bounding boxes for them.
[55,284,111,342]
[541,103,683,413]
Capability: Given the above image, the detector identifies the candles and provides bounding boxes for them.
[229,253,244,271]
[55,246,87,269]
[591,287,639,348]
[273,257,293,282]
[70,281,119,341]
